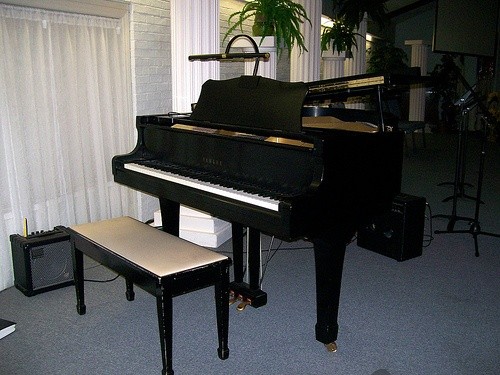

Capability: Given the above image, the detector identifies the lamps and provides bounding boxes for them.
[188,34,271,76]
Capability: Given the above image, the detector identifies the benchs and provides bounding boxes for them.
[65,214,233,375]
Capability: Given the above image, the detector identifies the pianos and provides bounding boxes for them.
[112,64,443,344]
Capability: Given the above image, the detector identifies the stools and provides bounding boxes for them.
[404,120,432,159]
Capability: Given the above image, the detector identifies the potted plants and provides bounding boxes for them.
[221,0,313,65]
[365,37,409,85]
[320,23,366,61]
